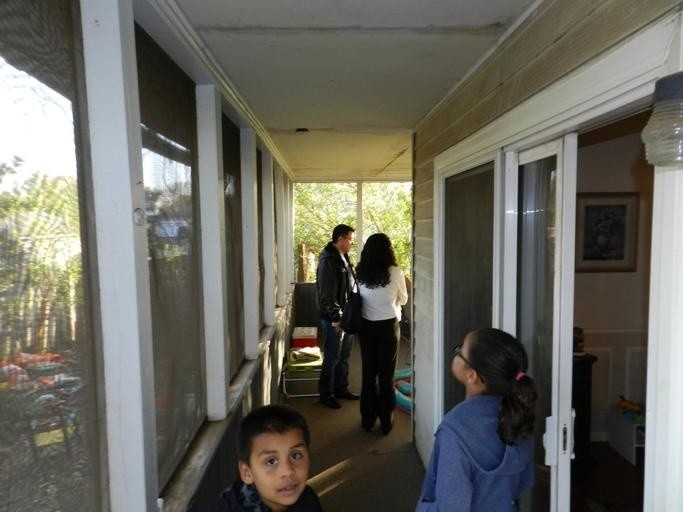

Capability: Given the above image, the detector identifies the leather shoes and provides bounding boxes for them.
[318,394,341,408]
[333,388,359,400]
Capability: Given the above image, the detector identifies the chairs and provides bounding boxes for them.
[289,282,327,335]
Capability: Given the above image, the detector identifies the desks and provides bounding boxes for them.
[281,351,323,400]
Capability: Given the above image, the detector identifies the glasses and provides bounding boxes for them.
[449,343,486,385]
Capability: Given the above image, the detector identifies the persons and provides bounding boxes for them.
[316,223,360,410]
[217,403,326,511]
[413,326,539,512]
[354,232,410,436]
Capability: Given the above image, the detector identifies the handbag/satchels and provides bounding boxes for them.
[339,289,362,335]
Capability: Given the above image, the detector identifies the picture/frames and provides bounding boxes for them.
[576,190,640,273]
